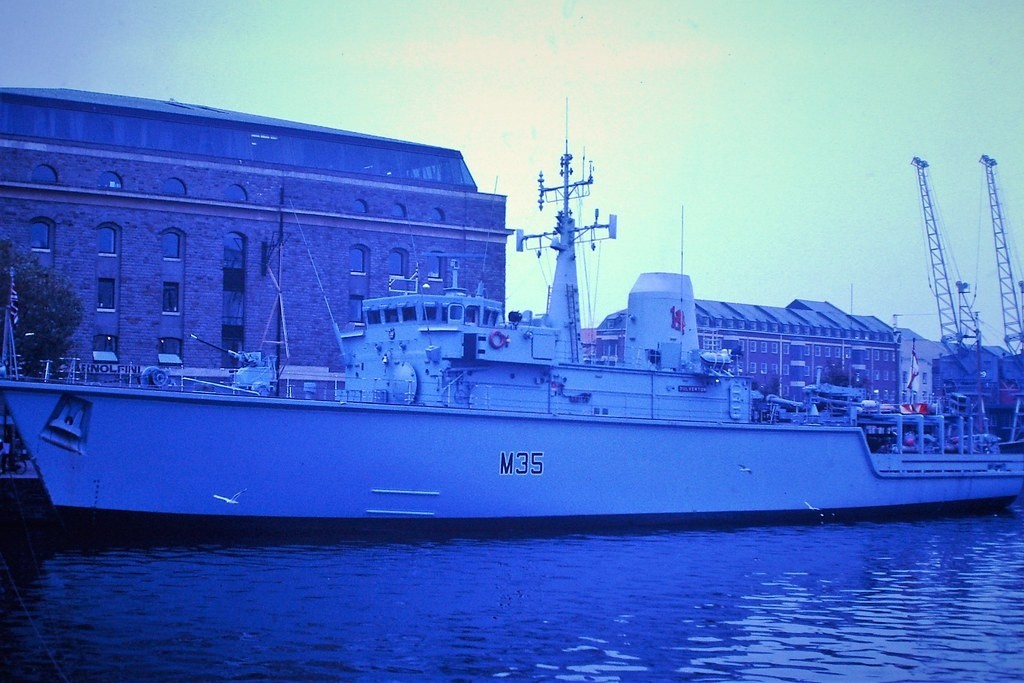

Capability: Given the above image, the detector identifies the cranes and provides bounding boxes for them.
[911,156,982,385]
[979,153,1024,365]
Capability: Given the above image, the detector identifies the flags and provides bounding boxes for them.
[907,350,920,395]
[9,277,19,332]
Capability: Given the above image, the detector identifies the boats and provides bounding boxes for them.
[0,95,1024,541]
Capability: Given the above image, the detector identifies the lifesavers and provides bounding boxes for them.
[488,328,506,350]
